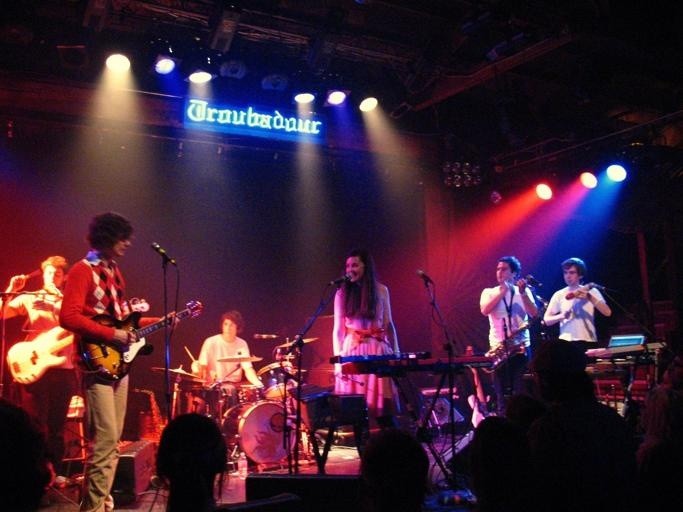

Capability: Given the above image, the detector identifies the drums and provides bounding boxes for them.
[174,384,211,416]
[241,384,258,401]
[206,382,239,415]
[221,397,296,464]
[263,383,290,405]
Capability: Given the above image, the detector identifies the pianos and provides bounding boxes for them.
[342,355,494,374]
[584,342,667,359]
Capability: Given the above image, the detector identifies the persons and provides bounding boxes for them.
[332,248,401,417]
[0,400,52,511]
[360,429,429,511]
[542,257,613,364]
[472,339,683,512]
[191,310,264,389]
[156,412,231,512]
[0,255,76,470]
[479,255,540,410]
[58,210,181,511]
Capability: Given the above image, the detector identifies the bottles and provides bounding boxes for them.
[237,451,248,481]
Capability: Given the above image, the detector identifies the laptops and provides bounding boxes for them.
[597,334,646,361]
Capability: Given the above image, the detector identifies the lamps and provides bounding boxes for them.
[143,13,415,121]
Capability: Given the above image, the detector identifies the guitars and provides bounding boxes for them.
[80,298,201,382]
[6,297,150,384]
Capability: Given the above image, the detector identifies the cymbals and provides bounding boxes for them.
[151,366,202,380]
[279,339,319,348]
[219,358,263,364]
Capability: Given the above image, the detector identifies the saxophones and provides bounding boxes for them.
[480,296,550,374]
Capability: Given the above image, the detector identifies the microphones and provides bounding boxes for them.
[329,274,352,286]
[566,283,595,300]
[416,269,435,286]
[527,275,544,289]
[151,242,178,268]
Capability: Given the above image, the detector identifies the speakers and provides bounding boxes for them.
[245,474,360,503]
